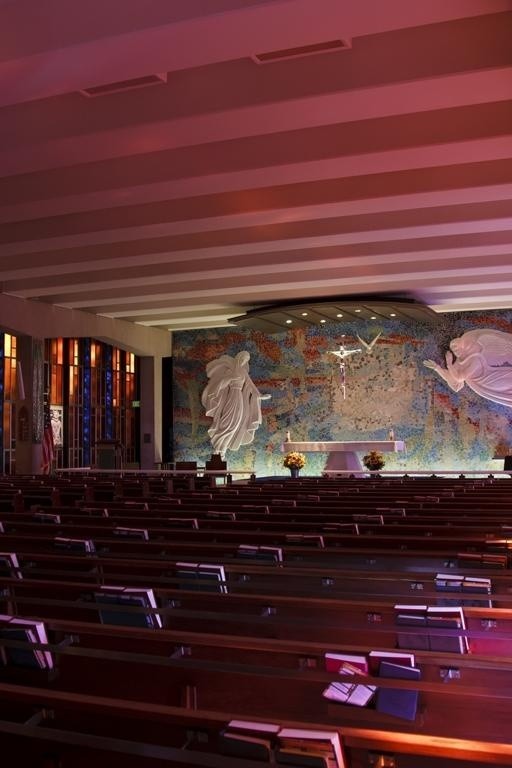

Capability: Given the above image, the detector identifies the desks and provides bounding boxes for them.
[281,441,404,477]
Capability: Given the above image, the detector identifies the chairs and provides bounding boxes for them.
[175,461,197,469]
[206,454,227,470]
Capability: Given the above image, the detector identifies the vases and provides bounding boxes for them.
[369,466,379,477]
[290,467,299,478]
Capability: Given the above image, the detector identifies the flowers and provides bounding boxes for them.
[284,452,304,469]
[363,452,384,467]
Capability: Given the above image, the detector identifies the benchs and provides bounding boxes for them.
[1,474,512,767]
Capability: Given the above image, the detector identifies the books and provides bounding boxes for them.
[84,508,108,517]
[458,551,508,568]
[435,573,493,611]
[228,720,280,746]
[35,514,61,525]
[323,523,359,535]
[208,511,235,520]
[327,652,420,721]
[281,731,344,768]
[1,553,22,577]
[319,491,338,497]
[124,503,148,511]
[376,507,405,516]
[51,537,93,557]
[160,498,182,504]
[175,563,229,594]
[170,517,198,529]
[235,544,282,560]
[193,493,212,500]
[353,514,384,524]
[272,500,297,507]
[286,534,325,547]
[242,505,269,513]
[299,496,319,502]
[96,585,161,628]
[394,605,467,655]
[116,528,149,540]
[0,614,52,669]
